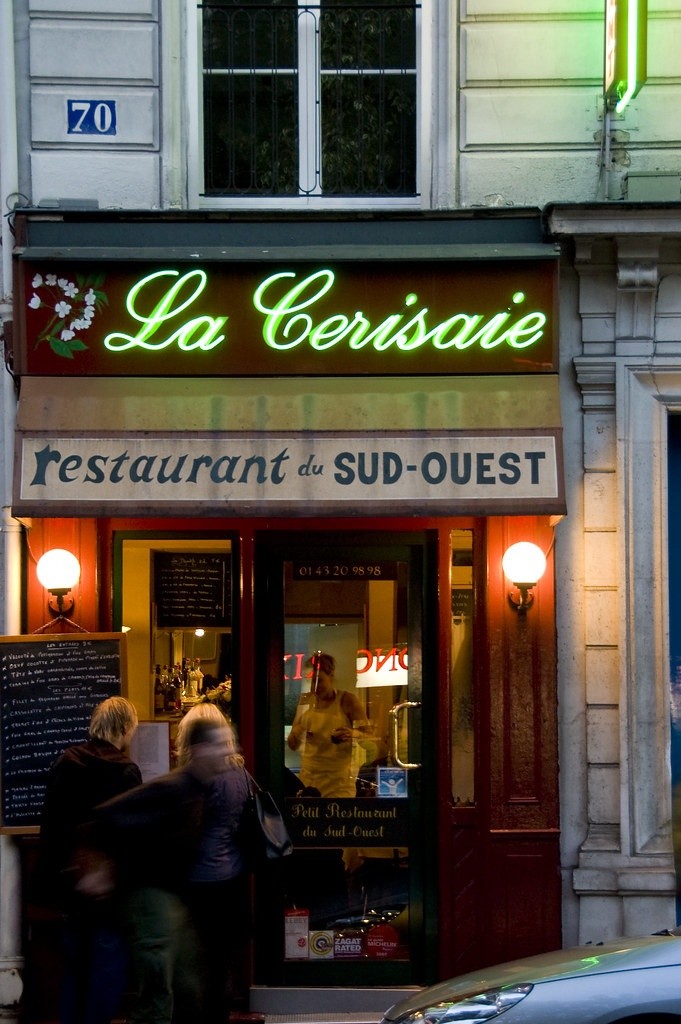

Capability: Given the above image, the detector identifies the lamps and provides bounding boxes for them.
[36,549,81,616]
[503,542,547,617]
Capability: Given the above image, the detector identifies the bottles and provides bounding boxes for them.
[155,656,204,716]
[300,696,320,756]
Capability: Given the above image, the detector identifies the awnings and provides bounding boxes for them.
[11,376,569,519]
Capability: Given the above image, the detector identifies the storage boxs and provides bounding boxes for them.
[308,930,334,959]
[285,909,309,959]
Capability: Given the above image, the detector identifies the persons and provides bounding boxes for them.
[288,654,371,798]
[39,694,253,1024]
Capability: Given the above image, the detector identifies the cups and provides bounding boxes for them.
[330,728,342,745]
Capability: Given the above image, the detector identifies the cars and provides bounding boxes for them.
[381,928,681,1024]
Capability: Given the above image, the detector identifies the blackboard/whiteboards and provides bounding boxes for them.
[152,550,234,633]
[0,632,129,834]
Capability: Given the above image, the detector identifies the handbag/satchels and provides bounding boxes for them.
[241,768,294,879]
